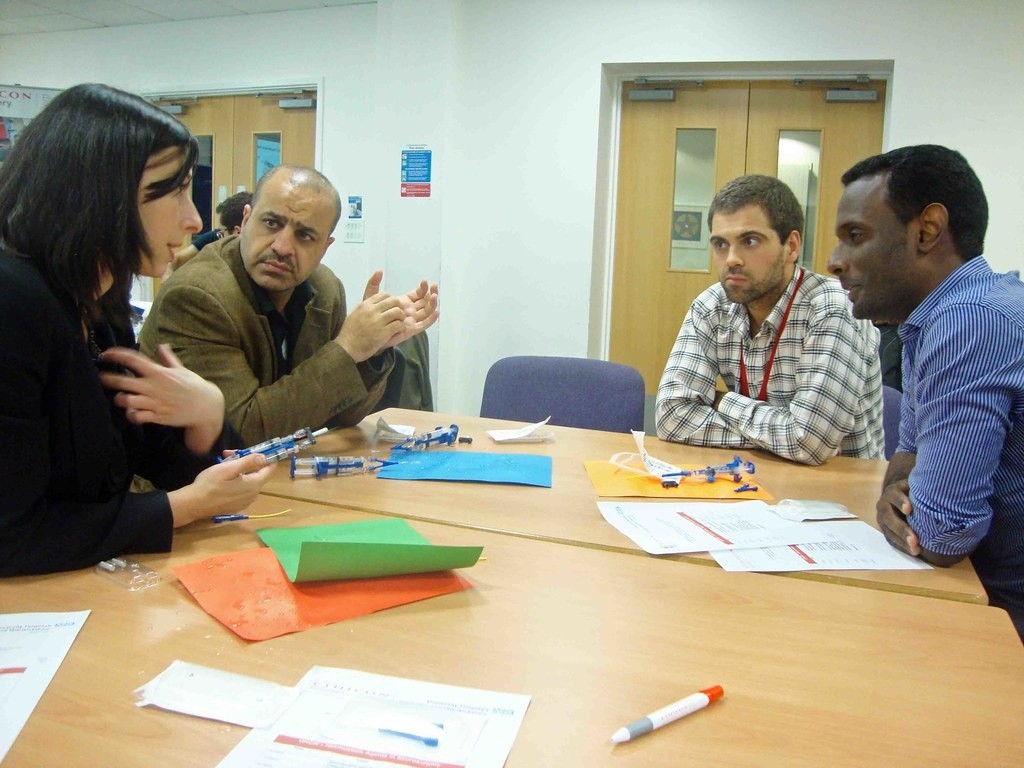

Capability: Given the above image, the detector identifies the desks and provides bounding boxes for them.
[0,406,1023,767]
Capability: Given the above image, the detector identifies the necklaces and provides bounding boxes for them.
[80,311,103,355]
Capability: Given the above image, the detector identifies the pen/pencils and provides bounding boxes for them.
[608,684,724,742]
[378,728,439,747]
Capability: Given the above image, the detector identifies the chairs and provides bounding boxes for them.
[479,355,645,435]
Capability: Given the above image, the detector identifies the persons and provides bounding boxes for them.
[828,145,1023,639]
[653,175,887,465]
[172,190,258,271]
[0,84,278,577]
[129,164,440,492]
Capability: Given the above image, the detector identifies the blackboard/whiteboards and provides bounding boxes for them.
[0,84,66,165]
